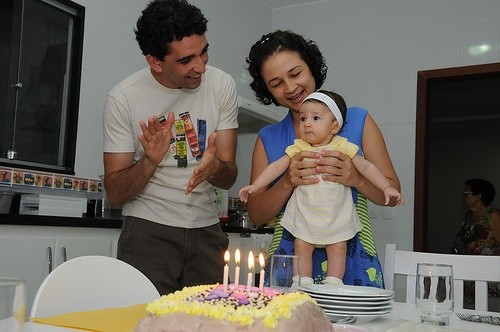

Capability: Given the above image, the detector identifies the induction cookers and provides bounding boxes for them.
[218,207,275,234]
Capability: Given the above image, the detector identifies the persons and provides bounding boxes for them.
[102,0,238,297]
[238,90,406,286]
[245,30,401,290]
[452,178,500,297]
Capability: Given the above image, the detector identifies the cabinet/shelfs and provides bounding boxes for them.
[0,224,115,321]
[225,232,274,286]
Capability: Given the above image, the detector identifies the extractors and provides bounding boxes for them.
[235,96,285,136]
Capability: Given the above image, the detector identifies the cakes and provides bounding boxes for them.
[135,282,335,332]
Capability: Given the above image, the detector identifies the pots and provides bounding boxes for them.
[234,211,253,226]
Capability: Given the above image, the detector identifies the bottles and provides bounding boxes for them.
[13,281,26,327]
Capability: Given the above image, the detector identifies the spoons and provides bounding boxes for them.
[330,316,356,325]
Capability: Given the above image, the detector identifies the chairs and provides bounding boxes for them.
[29,255,161,322]
[383,244,500,313]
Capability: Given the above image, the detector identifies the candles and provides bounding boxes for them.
[223,249,231,291]
[247,250,255,293]
[259,253,265,291]
[234,249,240,286]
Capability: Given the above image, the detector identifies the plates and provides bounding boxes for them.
[298,285,395,324]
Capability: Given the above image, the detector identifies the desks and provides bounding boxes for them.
[0,286,500,332]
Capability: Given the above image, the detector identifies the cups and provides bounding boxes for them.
[415,263,455,326]
[270,255,301,293]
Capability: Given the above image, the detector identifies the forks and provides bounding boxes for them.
[455,313,500,325]
